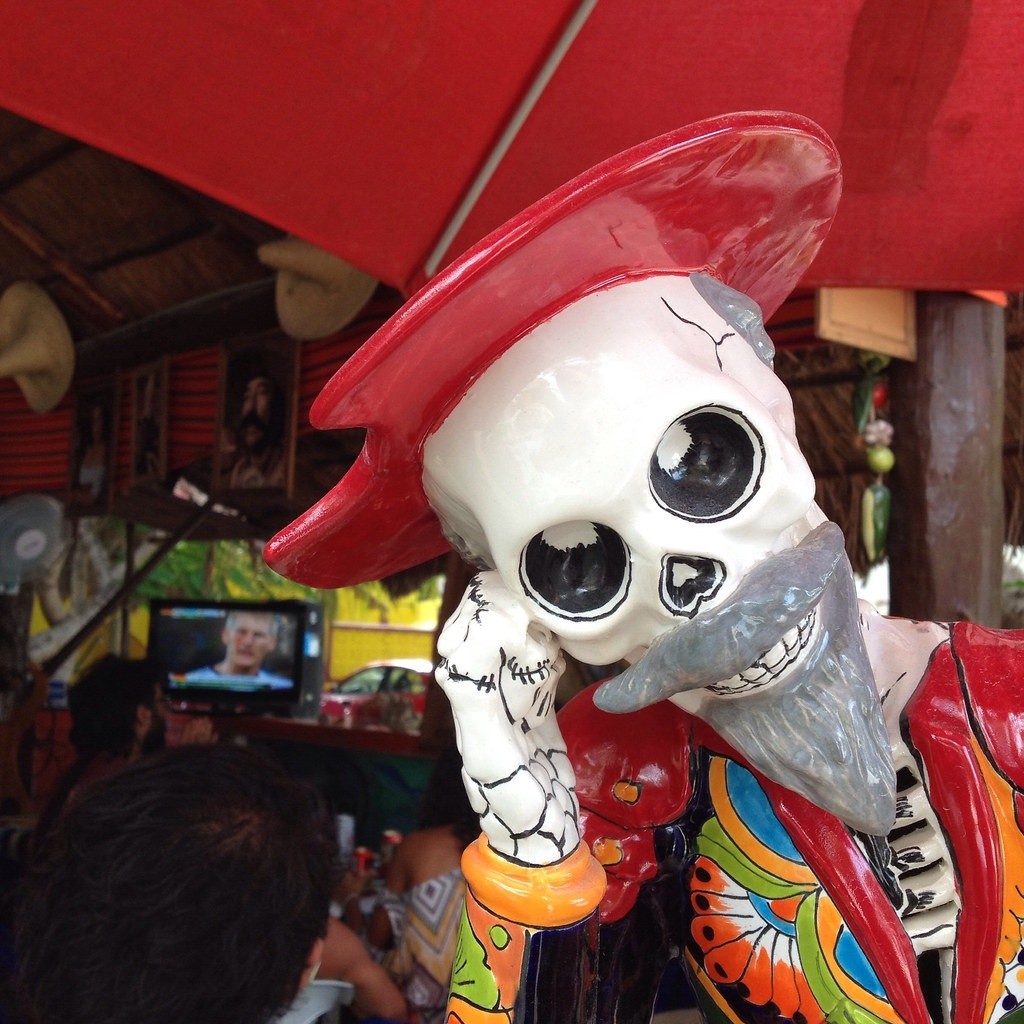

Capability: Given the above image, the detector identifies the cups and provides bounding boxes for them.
[389,867,463,1008]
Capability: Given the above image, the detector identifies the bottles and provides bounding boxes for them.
[336,828,415,952]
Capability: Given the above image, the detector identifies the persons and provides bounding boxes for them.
[332,735,482,1024]
[1,737,328,1024]
[174,610,297,692]
[33,650,220,830]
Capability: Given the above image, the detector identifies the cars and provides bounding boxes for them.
[317,659,432,733]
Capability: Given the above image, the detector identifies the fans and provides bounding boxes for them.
[0,495,68,597]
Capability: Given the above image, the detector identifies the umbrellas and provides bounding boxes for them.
[0,0,1024,310]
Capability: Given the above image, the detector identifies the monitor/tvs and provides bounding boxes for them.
[143,597,323,719]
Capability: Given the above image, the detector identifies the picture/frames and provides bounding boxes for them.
[127,359,172,485]
[211,330,300,497]
[68,369,120,518]
